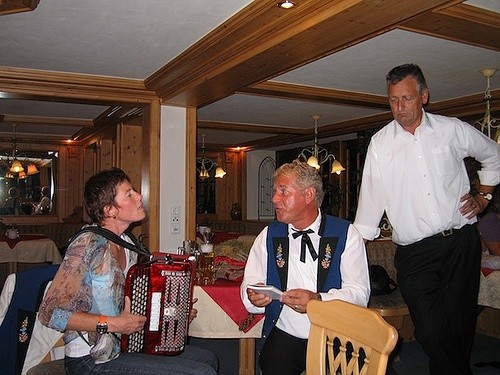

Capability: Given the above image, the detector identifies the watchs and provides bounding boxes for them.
[475,190,493,201]
[96,315,108,335]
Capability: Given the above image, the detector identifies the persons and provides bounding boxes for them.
[351,63,500,375]
[39,167,218,375]
[35,186,51,214]
[5,187,21,208]
[240,159,371,375]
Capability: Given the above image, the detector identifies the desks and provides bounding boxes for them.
[0,234,64,272]
[196,230,245,245]
[188,279,266,375]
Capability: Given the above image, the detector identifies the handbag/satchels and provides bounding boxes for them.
[368,264,398,296]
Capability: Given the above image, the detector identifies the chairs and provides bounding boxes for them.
[305,300,398,375]
[368,240,414,341]
[0,266,66,375]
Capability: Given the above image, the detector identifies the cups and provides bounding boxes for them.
[8,230,16,239]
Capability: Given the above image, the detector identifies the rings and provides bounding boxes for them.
[295,305,298,310]
[471,204,476,208]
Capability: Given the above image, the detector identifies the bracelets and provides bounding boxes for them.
[316,292,322,301]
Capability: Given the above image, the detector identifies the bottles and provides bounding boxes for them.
[197,244,215,286]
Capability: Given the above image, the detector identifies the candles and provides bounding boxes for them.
[201,243,213,253]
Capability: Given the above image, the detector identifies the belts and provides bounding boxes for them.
[418,223,477,243]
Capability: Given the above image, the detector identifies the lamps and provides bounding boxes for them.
[473,68,500,144]
[5,124,40,179]
[197,133,227,182]
[298,116,346,175]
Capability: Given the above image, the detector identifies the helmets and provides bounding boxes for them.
[40,186,50,194]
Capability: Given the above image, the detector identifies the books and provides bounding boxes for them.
[247,284,290,300]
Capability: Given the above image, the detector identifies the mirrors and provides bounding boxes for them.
[0,150,59,218]
